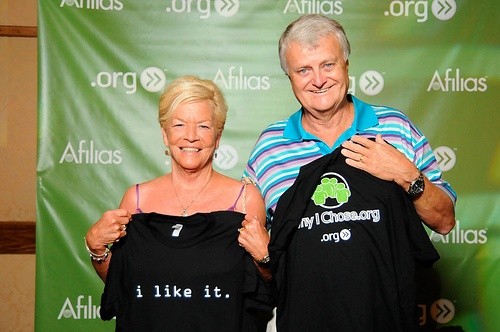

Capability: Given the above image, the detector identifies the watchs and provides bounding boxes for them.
[406,171,425,199]
[255,255,270,263]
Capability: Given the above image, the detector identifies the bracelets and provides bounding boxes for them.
[84,237,110,261]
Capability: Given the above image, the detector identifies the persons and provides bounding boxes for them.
[84,76,274,331]
[240,13,458,332]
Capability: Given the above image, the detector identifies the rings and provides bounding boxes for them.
[240,228,244,233]
[122,225,126,231]
[360,156,365,163]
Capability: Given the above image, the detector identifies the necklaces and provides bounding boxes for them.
[170,168,214,216]
